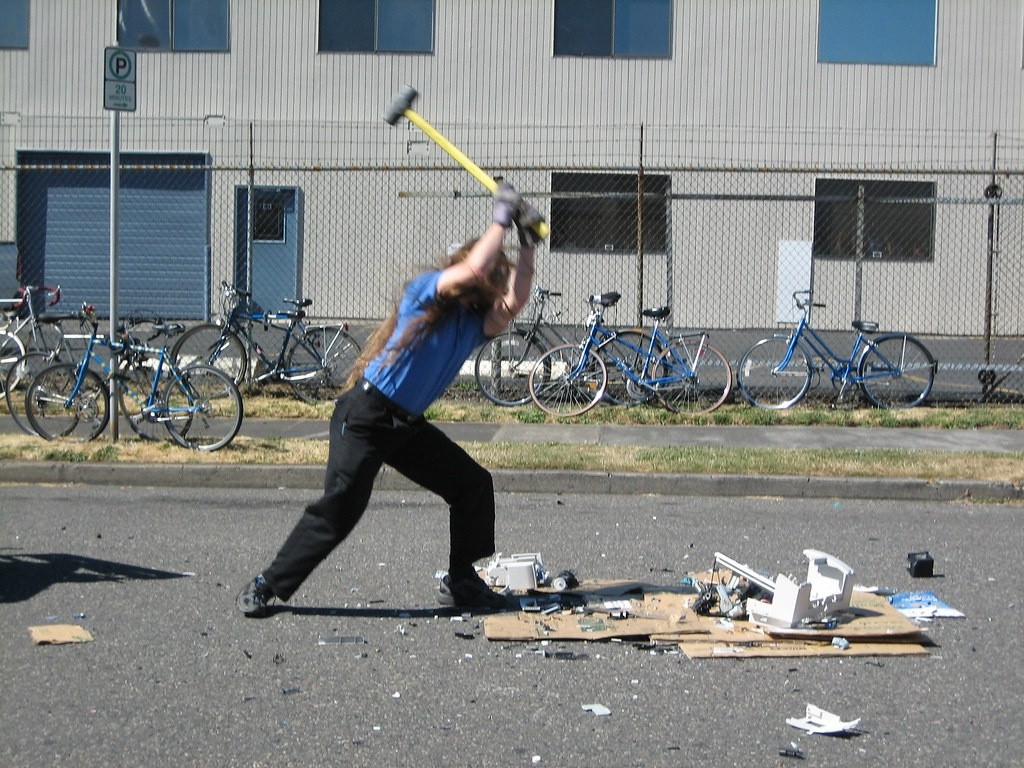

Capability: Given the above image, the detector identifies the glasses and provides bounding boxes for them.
[487,270,509,296]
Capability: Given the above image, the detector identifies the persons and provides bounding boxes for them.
[236,183,545,615]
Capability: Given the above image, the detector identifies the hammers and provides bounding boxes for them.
[380,86,550,248]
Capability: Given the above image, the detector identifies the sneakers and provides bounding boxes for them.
[235,577,276,613]
[438,574,507,609]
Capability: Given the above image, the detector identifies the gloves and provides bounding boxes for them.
[493,182,521,227]
[517,203,545,248]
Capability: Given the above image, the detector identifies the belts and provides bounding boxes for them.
[362,380,422,425]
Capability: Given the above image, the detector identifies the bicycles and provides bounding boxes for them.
[528,294,734,417]
[0,279,363,446]
[24,300,244,452]
[735,288,938,410]
[475,285,670,407]
